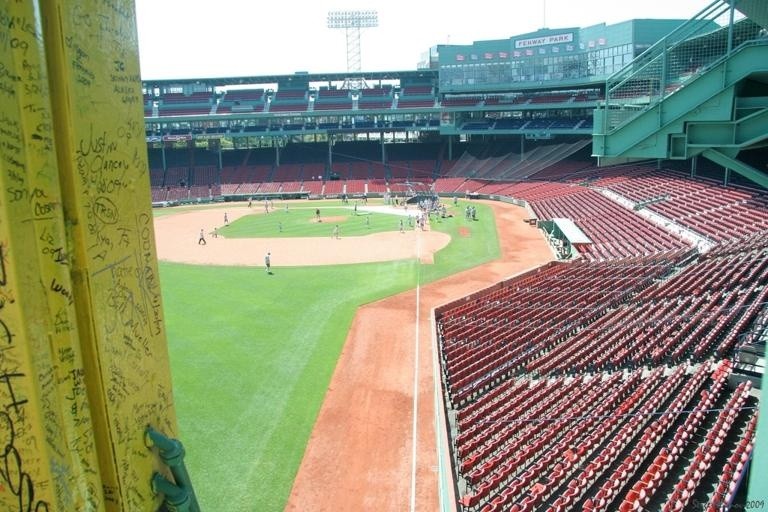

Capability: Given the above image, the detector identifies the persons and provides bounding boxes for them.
[341,192,345,204]
[269,198,273,209]
[278,222,282,232]
[264,201,269,214]
[352,202,358,215]
[345,194,349,204]
[264,252,272,273]
[198,229,206,244]
[285,204,289,213]
[246,196,252,208]
[364,196,368,206]
[362,216,371,229]
[223,212,229,226]
[331,225,341,239]
[380,192,477,234]
[361,198,365,206]
[315,207,321,221]
[211,227,218,238]
